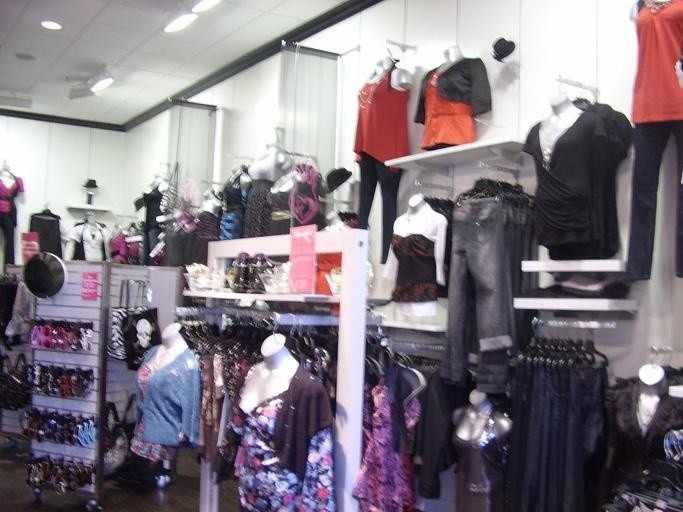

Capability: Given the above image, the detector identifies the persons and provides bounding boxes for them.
[235,332,331,511]
[142,172,168,265]
[0,164,24,263]
[523,94,605,244]
[609,364,683,461]
[632,0,682,128]
[355,57,413,265]
[246,143,285,237]
[220,163,251,240]
[74,213,107,262]
[390,193,448,304]
[128,324,202,476]
[416,47,493,149]
[198,190,221,241]
[450,390,514,511]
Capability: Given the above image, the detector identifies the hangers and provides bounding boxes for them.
[454,161,531,208]
[517,316,608,371]
[173,307,325,363]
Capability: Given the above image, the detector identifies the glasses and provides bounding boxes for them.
[26,362,95,397]
[25,456,95,494]
[27,317,94,353]
[24,409,97,446]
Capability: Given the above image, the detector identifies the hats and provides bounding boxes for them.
[491,38,514,62]
[326,167,352,193]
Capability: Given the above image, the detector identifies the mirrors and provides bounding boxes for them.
[23,253,66,299]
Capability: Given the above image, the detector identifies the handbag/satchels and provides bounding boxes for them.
[105,278,150,361]
[122,280,162,371]
[103,402,128,474]
[0,353,32,410]
[121,393,142,460]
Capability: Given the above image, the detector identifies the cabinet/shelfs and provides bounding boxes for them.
[26,262,185,499]
